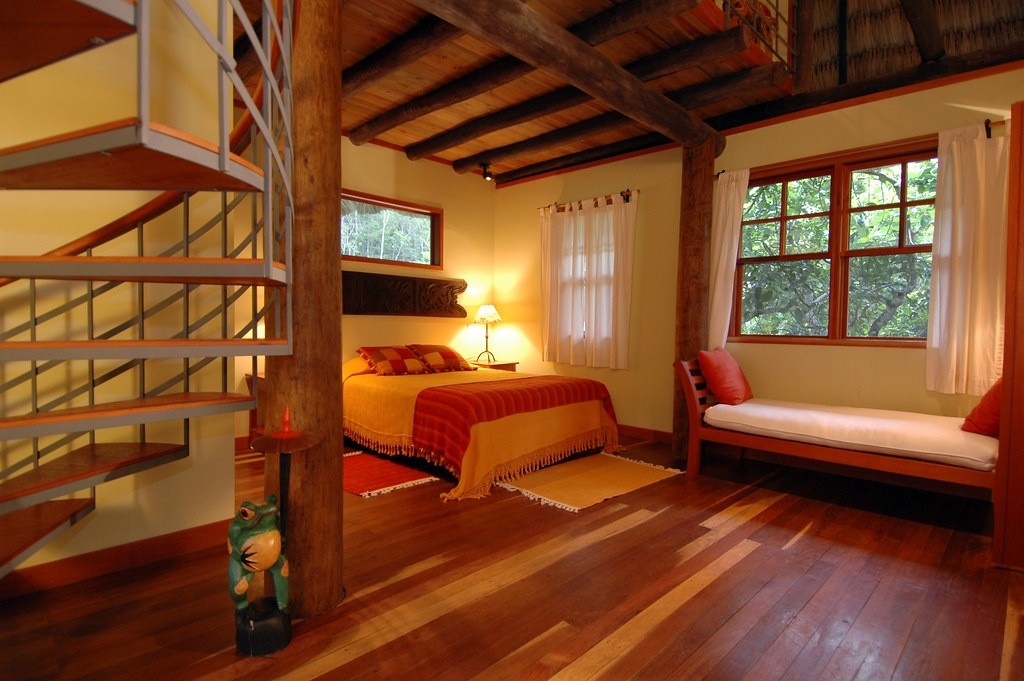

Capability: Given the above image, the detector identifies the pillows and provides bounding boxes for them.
[960,376,1003,437]
[696,345,754,406]
[405,344,479,373]
[355,346,432,376]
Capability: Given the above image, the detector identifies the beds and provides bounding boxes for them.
[342,357,626,504]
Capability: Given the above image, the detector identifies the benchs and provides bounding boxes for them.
[673,357,1007,566]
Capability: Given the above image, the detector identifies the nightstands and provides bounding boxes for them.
[471,361,519,372]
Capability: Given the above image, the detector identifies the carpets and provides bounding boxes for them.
[343,451,440,499]
[496,451,687,513]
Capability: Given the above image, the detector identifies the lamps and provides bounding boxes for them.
[473,304,501,362]
[479,163,492,181]
